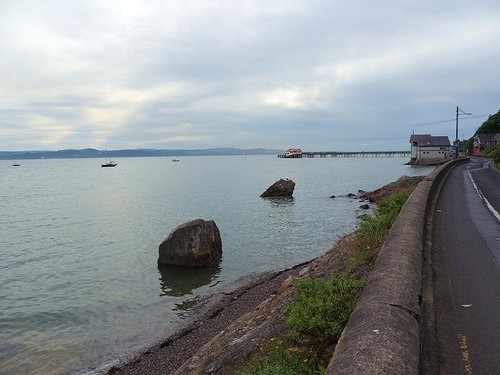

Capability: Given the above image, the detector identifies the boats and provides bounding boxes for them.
[173,159,180,162]
[13,164,20,166]
[101,155,118,167]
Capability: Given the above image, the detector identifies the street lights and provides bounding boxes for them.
[455,105,472,159]
